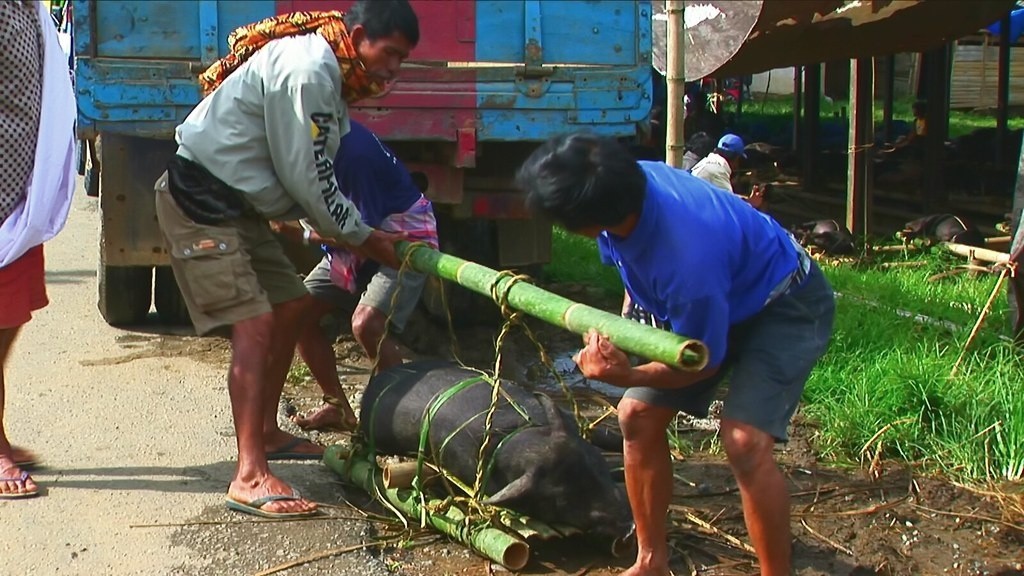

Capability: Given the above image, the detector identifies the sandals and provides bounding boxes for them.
[0,455,40,497]
[10,445,43,465]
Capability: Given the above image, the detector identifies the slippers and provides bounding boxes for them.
[266,437,329,459]
[227,486,318,518]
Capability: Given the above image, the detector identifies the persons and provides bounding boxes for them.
[154,0,441,517]
[682,90,765,208]
[887,102,931,153]
[1,0,81,499]
[518,129,836,576]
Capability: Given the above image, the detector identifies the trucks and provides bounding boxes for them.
[65,0,655,328]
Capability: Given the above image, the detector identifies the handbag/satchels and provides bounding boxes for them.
[166,152,259,224]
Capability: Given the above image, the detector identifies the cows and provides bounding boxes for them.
[360,359,637,543]
[903,212,985,249]
[791,219,858,255]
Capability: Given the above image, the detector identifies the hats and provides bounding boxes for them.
[717,134,748,158]
